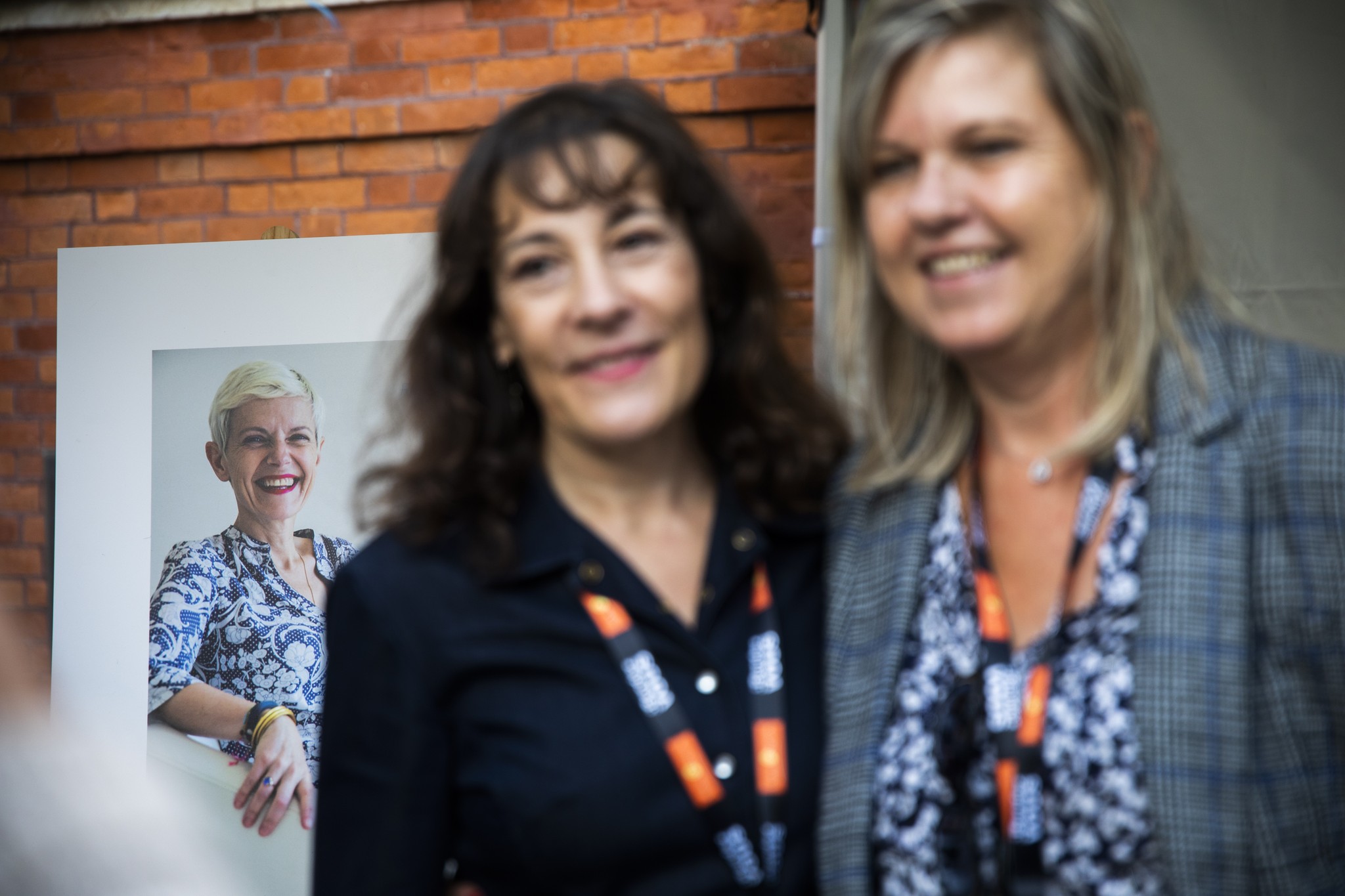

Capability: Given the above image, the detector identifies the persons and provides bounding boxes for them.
[301,77,851,895]
[800,0,1345,895]
[154,359,363,838]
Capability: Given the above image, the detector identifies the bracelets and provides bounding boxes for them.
[236,698,297,749]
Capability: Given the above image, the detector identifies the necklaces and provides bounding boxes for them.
[983,421,1105,486]
[268,550,316,609]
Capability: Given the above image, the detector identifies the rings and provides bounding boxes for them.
[262,777,275,787]
